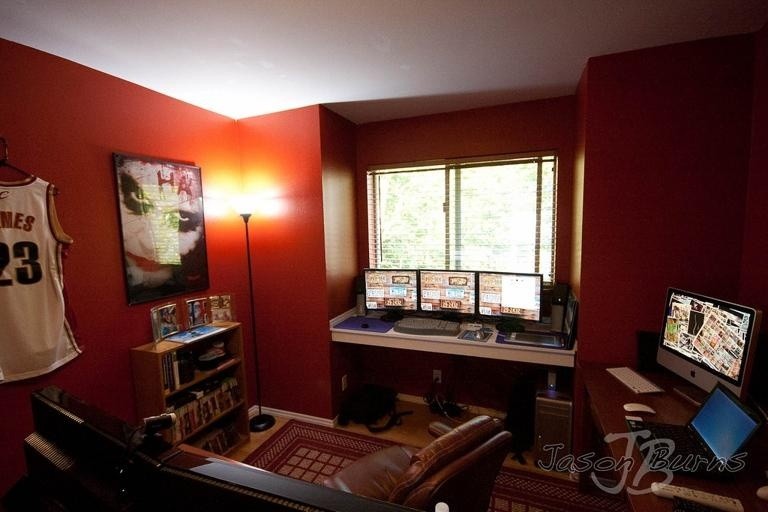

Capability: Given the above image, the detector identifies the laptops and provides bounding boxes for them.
[625,380,764,476]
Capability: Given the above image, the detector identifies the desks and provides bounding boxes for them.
[330,311,574,369]
[580,356,768,511]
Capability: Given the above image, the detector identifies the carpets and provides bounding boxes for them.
[239,418,630,511]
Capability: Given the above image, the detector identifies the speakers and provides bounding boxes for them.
[550,285,568,333]
[355,278,366,317]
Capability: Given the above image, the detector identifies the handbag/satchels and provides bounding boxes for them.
[338,385,399,433]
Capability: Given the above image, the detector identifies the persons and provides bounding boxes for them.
[116,157,205,291]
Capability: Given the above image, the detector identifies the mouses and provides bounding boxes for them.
[474,328,486,340]
[622,401,656,415]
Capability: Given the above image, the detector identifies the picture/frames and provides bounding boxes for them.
[110,152,211,306]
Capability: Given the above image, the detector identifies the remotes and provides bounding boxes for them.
[651,481,747,512]
[674,495,722,511]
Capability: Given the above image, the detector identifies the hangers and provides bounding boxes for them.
[0,137,60,195]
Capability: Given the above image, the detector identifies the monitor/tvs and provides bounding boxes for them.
[418,268,477,323]
[22,382,436,512]
[475,272,543,331]
[656,285,763,407]
[363,268,418,323]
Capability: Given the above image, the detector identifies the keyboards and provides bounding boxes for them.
[607,365,665,398]
[394,316,461,336]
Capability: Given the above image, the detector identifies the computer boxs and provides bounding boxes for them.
[534,386,574,473]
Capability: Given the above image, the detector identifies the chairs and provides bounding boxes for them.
[322,414,514,512]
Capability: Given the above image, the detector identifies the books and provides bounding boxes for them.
[149,303,177,342]
[165,325,228,345]
[209,295,233,323]
[163,351,182,394]
[186,297,209,328]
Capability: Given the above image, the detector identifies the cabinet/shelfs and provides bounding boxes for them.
[129,320,251,456]
